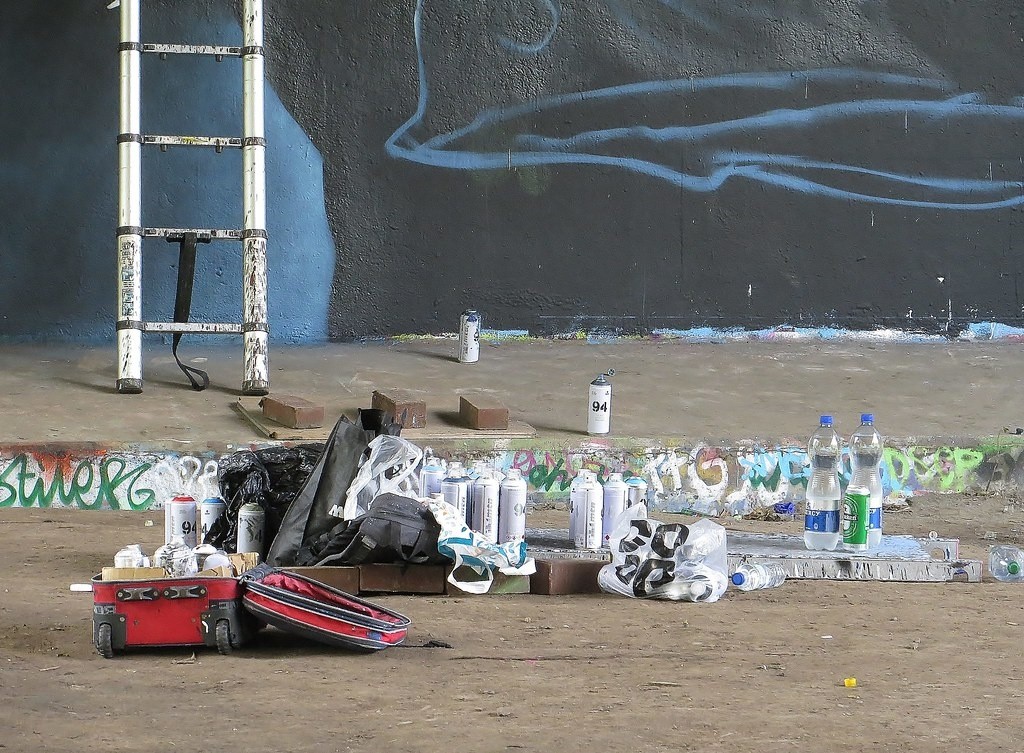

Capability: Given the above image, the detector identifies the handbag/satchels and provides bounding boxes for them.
[596,500,728,602]
[423,496,537,595]
[266,404,405,567]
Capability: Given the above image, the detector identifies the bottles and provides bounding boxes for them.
[732,562,786,591]
[458,308,481,364]
[849,414,884,548]
[237,500,265,559]
[568,468,648,552]
[989,545,1024,583]
[419,458,527,545]
[115,493,234,577]
[587,368,616,434]
[596,530,728,602]
[803,416,841,551]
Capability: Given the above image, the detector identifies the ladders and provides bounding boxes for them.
[115,0,271,396]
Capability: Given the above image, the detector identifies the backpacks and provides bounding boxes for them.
[293,493,452,567]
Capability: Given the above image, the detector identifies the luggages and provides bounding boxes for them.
[92,560,411,656]
